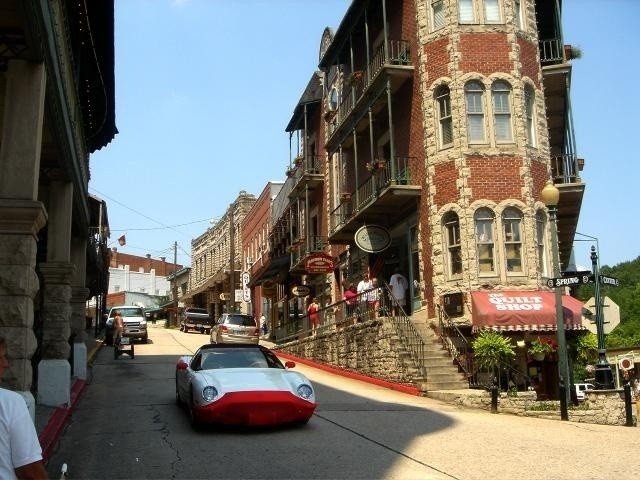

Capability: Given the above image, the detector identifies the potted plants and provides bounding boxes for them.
[526,339,552,362]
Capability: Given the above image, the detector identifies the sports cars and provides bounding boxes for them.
[175,343,317,435]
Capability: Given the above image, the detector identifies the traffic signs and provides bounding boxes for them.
[547,272,592,293]
[601,275,625,289]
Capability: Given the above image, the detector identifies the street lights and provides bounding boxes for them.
[541,181,574,417]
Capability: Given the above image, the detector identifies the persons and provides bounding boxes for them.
[0,335,50,480]
[259,313,266,335]
[307,297,320,335]
[111,310,125,345]
[323,269,410,328]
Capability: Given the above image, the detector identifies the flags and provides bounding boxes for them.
[118,235,126,246]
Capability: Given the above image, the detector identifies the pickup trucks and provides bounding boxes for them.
[180,306,213,334]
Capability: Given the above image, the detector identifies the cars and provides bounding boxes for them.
[575,383,595,401]
[106,306,148,342]
[209,314,259,346]
